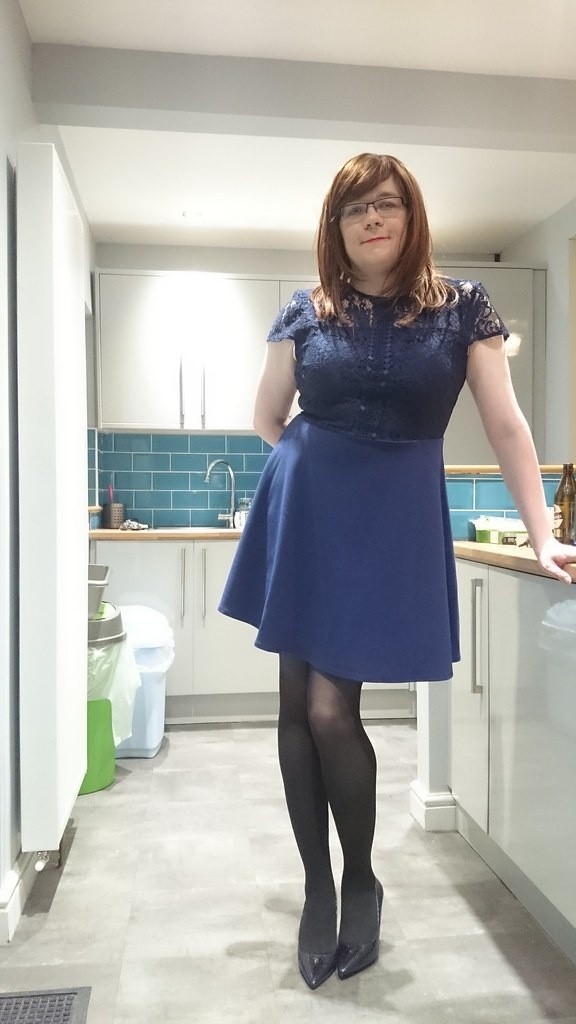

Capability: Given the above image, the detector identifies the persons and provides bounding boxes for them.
[218,153,576,990]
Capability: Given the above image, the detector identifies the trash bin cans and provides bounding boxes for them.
[79,598,126,795]
[115,646,169,759]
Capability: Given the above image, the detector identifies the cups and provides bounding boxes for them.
[238,498,251,511]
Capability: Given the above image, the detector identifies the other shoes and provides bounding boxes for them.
[331,871,383,982]
[298,884,339,988]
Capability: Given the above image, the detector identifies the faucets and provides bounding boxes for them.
[204,459,235,529]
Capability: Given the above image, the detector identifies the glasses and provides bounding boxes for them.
[336,193,408,221]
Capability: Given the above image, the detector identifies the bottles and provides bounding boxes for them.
[554,464,576,545]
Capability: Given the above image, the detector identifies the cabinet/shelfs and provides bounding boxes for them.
[89,539,417,725]
[423,261,547,466]
[280,275,352,426]
[448,556,575,928]
[94,266,280,437]
